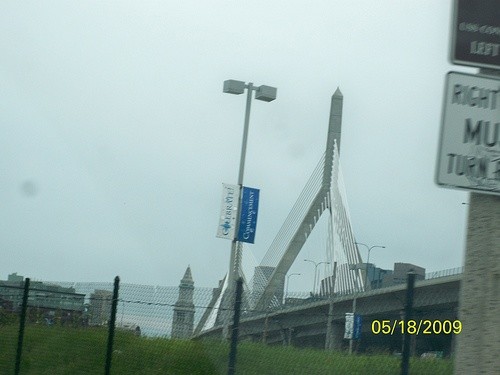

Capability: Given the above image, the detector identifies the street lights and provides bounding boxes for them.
[223,79,277,322]
[285,273,301,297]
[304,259,330,298]
[355,242,386,290]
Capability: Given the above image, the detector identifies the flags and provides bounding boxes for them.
[236,186,260,244]
[353,313,362,339]
[216,181,240,241]
[344,312,354,339]
